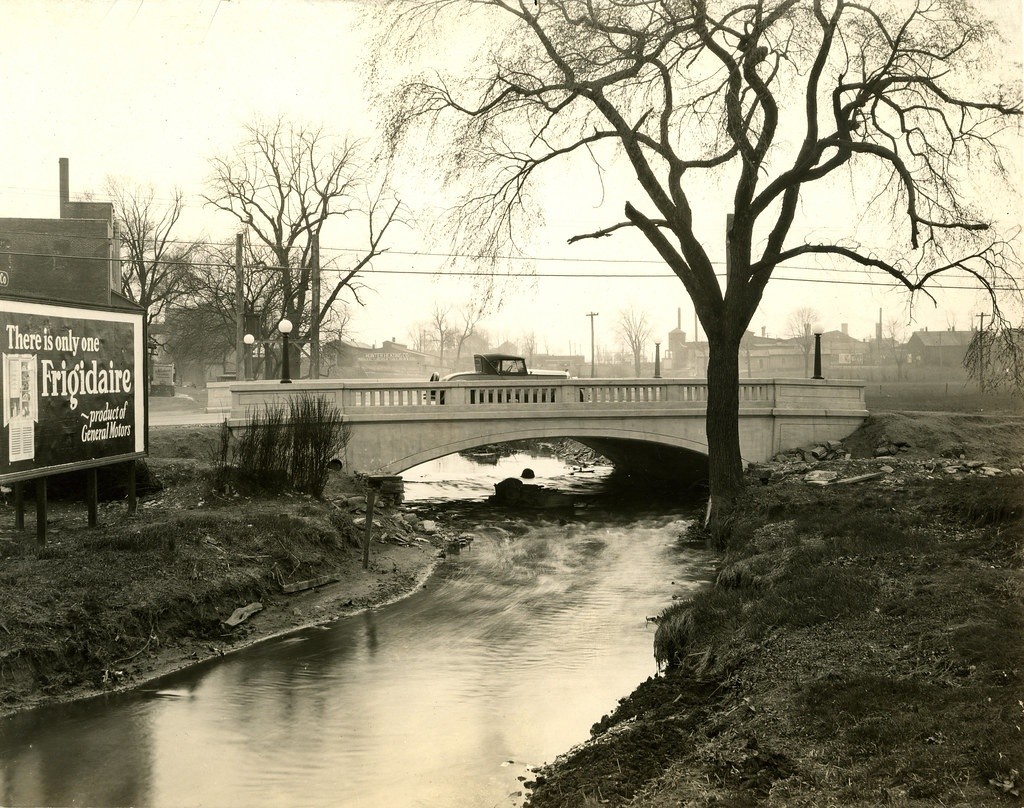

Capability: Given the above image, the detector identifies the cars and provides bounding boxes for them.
[422,352,584,403]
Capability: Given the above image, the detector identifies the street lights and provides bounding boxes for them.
[243,334,255,381]
[277,318,293,384]
[810,320,824,379]
[653,336,662,378]
